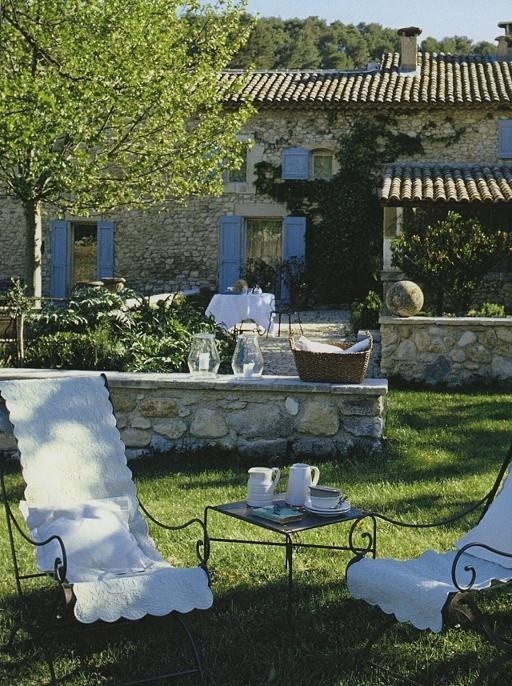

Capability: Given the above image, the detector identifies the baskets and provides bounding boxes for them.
[288,329,373,384]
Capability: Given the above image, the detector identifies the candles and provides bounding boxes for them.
[243,363,256,377]
[198,353,210,370]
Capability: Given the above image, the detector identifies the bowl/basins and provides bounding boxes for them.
[307,485,340,509]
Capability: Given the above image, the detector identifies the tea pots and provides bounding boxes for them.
[286,463,320,507]
[246,467,281,508]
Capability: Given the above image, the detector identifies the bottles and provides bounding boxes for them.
[231,332,265,382]
[188,334,220,379]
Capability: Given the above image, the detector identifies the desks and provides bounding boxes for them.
[204,493,377,637]
[204,292,275,333]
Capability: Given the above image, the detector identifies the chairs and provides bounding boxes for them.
[344,441,512,686]
[265,290,304,336]
[0,372,214,686]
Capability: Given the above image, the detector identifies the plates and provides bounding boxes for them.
[303,500,351,518]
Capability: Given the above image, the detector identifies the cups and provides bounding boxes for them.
[241,288,253,295]
[272,499,287,515]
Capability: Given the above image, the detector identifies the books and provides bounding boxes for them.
[251,506,303,524]
